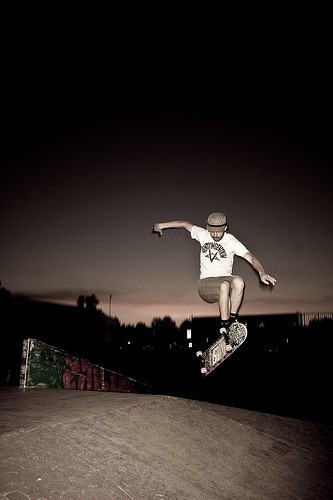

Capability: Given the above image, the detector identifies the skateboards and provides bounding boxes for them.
[196,322,248,377]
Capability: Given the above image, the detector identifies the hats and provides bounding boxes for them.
[206,212,227,232]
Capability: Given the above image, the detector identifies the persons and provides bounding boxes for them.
[153,212,277,335]
